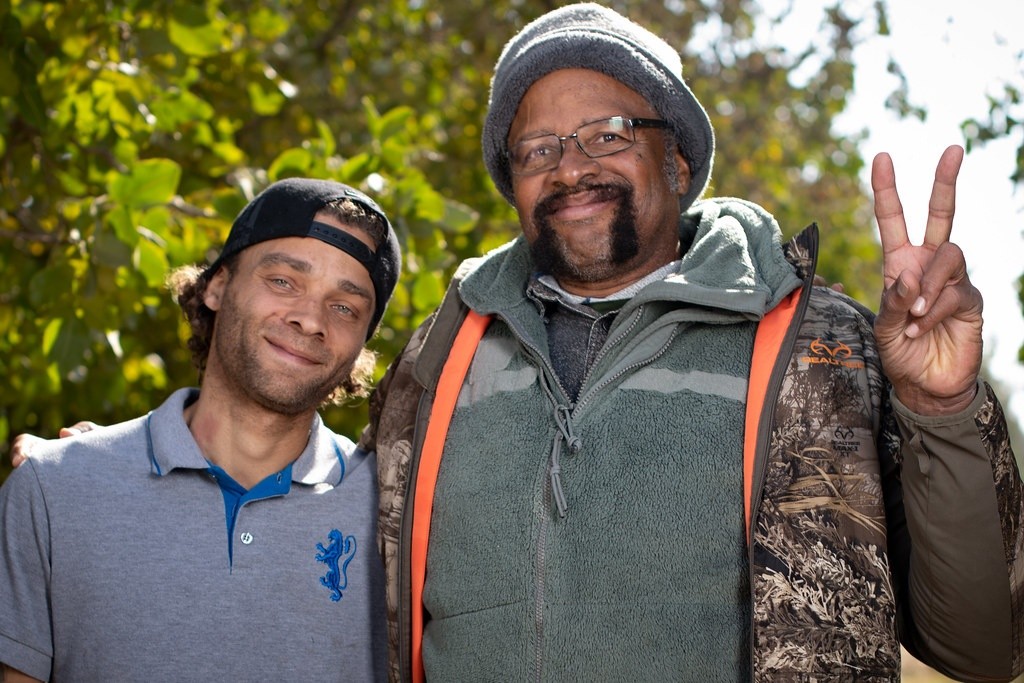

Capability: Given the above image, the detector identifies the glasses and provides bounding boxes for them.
[504,113,673,176]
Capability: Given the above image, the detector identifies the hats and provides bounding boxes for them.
[480,1,717,217]
[195,176,403,343]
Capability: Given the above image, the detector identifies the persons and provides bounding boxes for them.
[12,0,1024,683]
[0,179,842,683]
